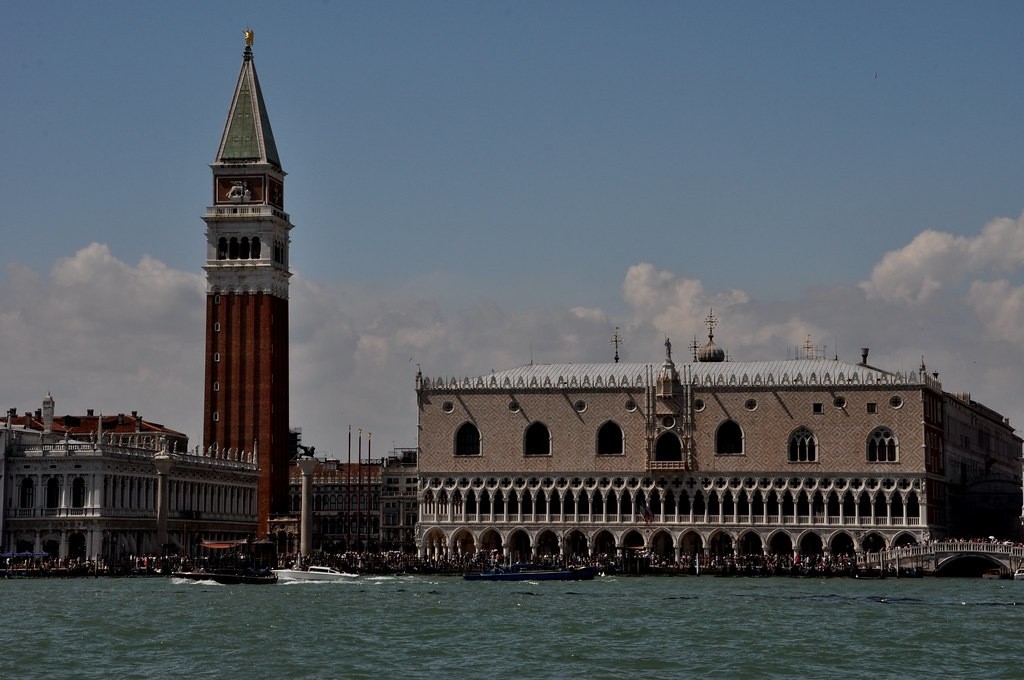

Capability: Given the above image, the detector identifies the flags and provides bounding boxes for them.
[634,500,654,523]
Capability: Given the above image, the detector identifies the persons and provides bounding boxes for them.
[0,537,1024,574]
[663,337,672,358]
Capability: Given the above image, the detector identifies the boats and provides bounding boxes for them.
[463,562,599,581]
[170,538,279,585]
[268,566,362,582]
[1013,569,1024,580]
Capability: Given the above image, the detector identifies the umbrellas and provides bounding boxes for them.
[253,539,274,544]
[0,551,14,564]
[15,550,33,563]
[33,550,51,563]
